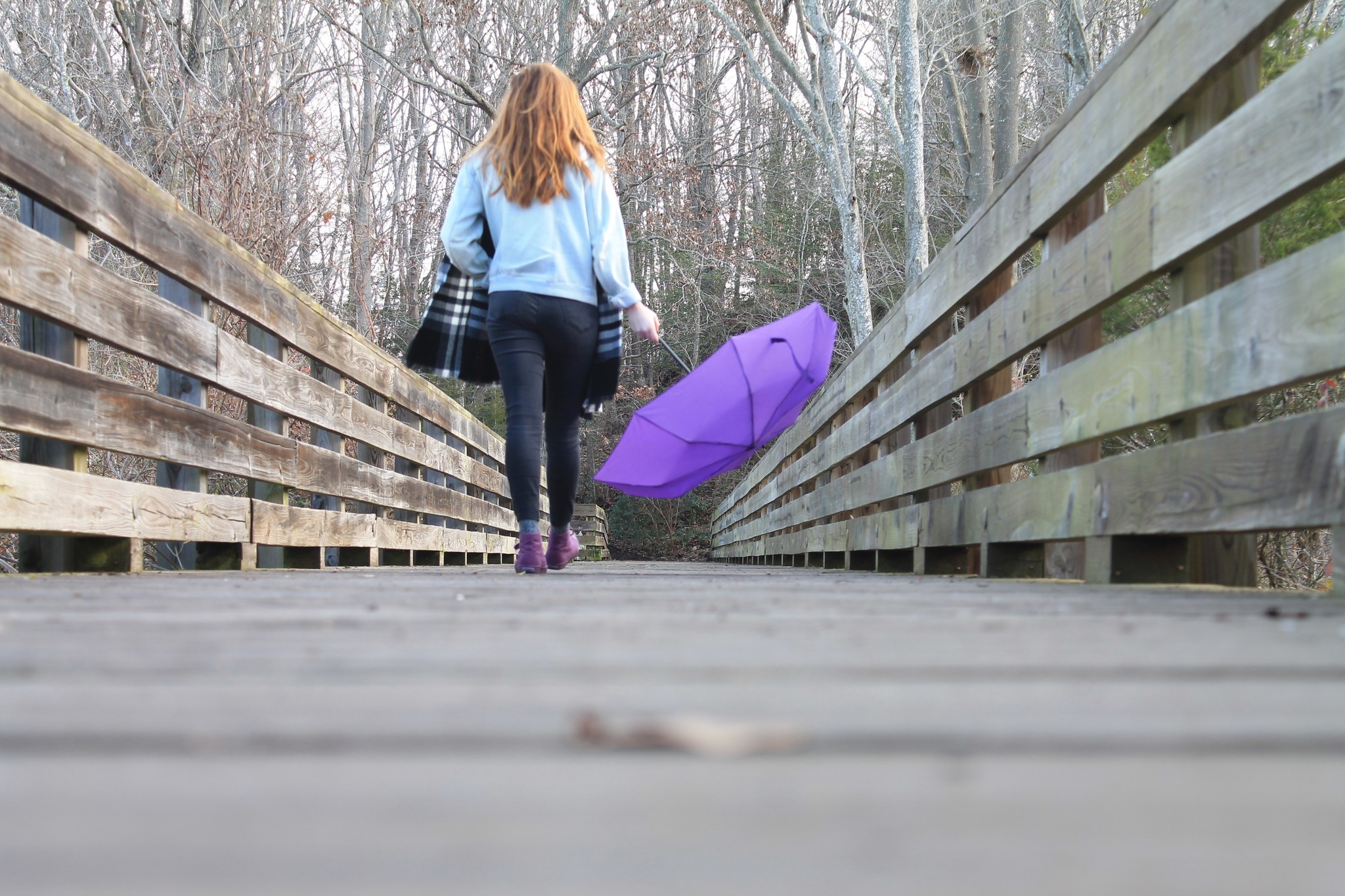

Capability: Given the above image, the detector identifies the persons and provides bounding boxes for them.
[439,62,662,574]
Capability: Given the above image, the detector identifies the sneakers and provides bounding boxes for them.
[545,528,579,570]
[515,532,548,574]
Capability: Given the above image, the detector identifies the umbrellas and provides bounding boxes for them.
[593,301,835,497]
